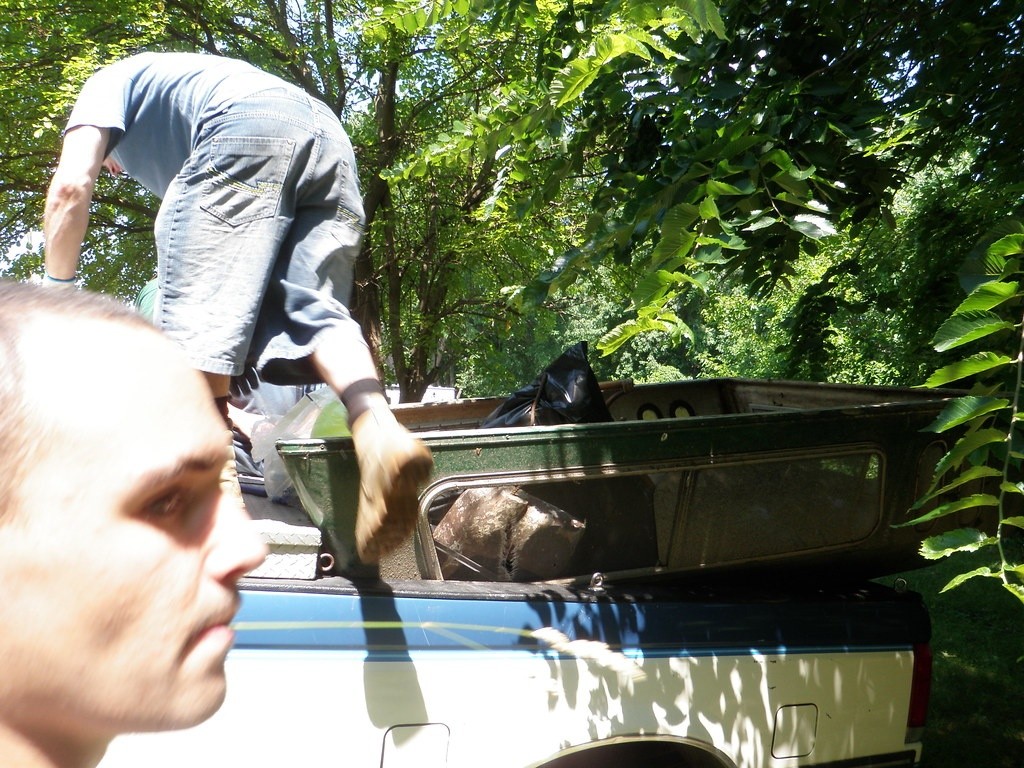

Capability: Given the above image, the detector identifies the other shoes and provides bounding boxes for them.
[354,431,435,566]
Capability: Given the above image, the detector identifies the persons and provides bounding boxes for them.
[42,51,434,563]
[0,277,267,768]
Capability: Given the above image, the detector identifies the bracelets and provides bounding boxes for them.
[45,271,76,283]
